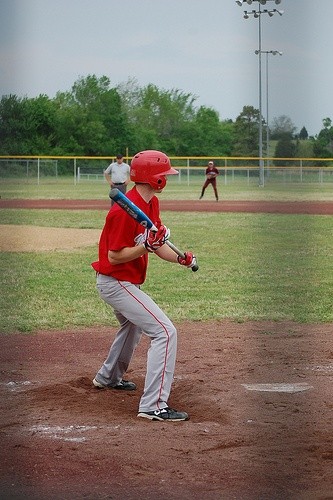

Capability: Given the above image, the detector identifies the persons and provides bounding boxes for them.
[105,154,130,207]
[91,151,197,422]
[200,161,219,201]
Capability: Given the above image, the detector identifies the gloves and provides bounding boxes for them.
[178,252,196,268]
[143,222,170,253]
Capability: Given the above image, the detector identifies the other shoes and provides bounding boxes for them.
[216,197,218,200]
[200,196,202,199]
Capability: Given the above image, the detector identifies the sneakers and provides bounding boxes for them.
[93,378,136,390]
[137,408,188,421]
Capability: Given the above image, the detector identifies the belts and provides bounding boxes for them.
[113,183,124,185]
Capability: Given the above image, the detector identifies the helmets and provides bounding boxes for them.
[130,150,179,190]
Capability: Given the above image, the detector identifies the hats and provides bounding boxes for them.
[209,161,213,164]
[117,154,122,159]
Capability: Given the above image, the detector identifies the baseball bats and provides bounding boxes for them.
[108,187,199,273]
